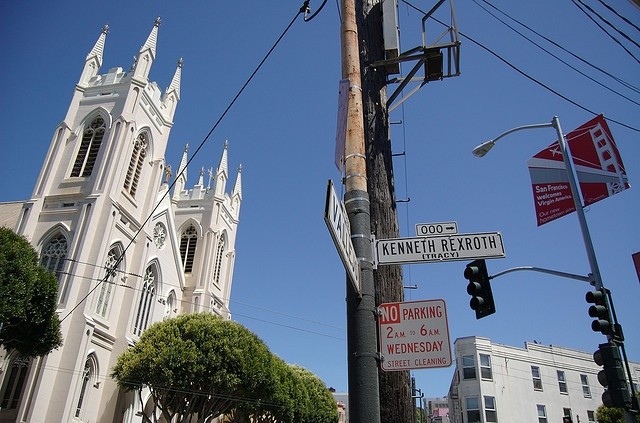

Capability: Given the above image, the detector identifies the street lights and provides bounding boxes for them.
[471,115,636,423]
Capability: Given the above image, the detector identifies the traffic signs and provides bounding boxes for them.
[376,231,506,266]
[326,179,364,299]
[415,221,458,236]
[525,114,631,227]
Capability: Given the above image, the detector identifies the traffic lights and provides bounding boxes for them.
[593,342,629,408]
[463,259,496,320]
[585,288,616,335]
